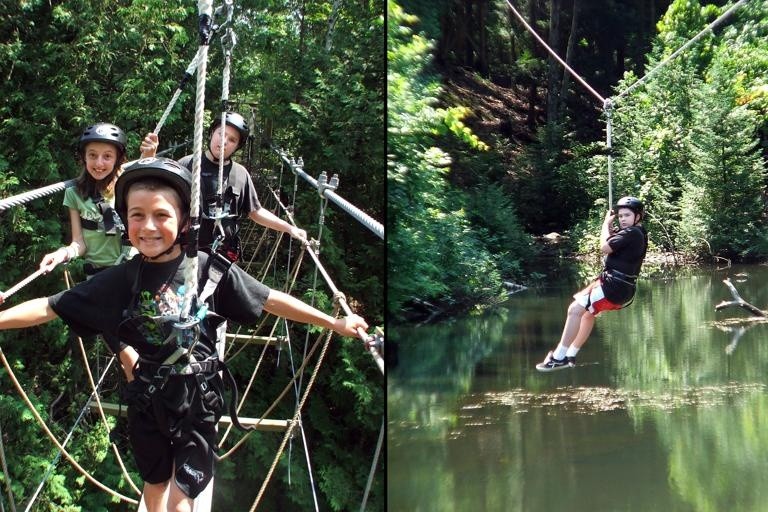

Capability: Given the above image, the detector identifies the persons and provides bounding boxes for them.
[170,112,307,263]
[38,122,160,386]
[0,159,370,511]
[534,196,647,370]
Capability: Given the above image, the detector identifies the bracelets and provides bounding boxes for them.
[59,247,72,265]
[68,245,77,261]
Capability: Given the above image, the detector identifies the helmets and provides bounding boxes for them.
[114,157,203,231]
[77,123,128,153]
[616,197,644,220]
[208,111,249,147]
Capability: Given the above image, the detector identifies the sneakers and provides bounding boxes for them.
[536,351,575,372]
